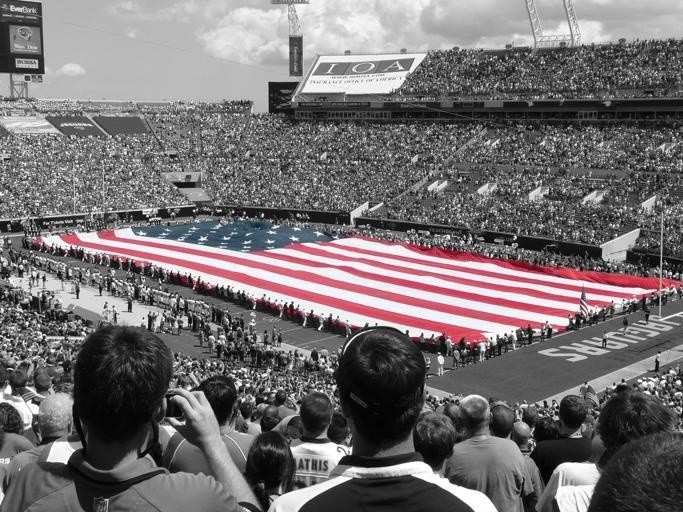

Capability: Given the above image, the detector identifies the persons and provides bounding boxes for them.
[445,394,533,512]
[568,300,615,331]
[656,351,661,371]
[247,294,378,346]
[0,96,681,281]
[267,326,498,512]
[149,345,353,511]
[312,38,683,103]
[406,328,496,378]
[497,320,552,356]
[0,235,120,472]
[116,256,258,364]
[623,284,683,315]
[1,326,263,511]
[489,365,683,512]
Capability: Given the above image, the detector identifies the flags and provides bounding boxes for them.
[580,291,588,317]
[33,219,683,349]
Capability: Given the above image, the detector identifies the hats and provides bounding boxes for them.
[336,327,425,409]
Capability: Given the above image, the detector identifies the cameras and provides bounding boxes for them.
[165,393,184,418]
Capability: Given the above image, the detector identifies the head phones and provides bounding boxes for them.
[489,404,517,423]
